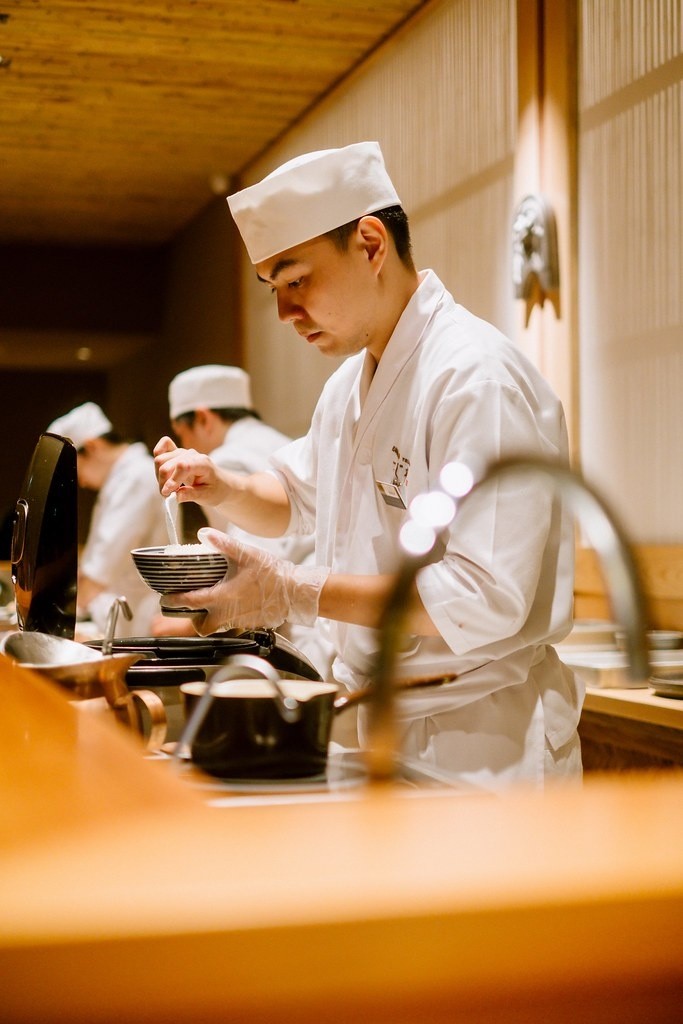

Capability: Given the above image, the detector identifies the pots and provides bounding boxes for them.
[178,652,457,782]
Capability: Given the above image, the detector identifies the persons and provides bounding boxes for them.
[45,402,183,638]
[149,364,333,681]
[153,141,586,792]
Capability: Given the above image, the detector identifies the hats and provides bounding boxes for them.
[168,364,253,420]
[45,401,114,450]
[226,141,402,265]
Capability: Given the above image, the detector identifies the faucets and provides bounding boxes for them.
[369,450,652,790]
[162,654,304,780]
[102,596,134,654]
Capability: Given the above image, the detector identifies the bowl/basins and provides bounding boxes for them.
[648,661,683,678]
[129,544,230,618]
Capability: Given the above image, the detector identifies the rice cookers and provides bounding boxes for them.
[8,431,261,743]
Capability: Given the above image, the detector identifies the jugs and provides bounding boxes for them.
[0,631,169,758]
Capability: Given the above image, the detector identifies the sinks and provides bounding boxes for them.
[81,636,260,657]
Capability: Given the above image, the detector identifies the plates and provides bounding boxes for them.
[646,675,683,699]
